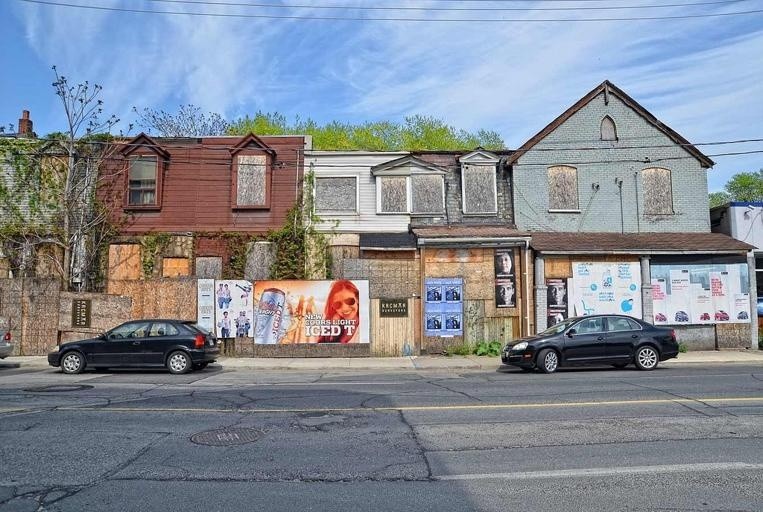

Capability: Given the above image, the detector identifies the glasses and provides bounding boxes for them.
[331,296,356,310]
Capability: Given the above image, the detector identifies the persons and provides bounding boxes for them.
[304,295,314,317]
[432,319,441,329]
[218,311,233,338]
[224,283,233,309]
[494,251,516,276]
[216,283,225,310]
[496,281,517,307]
[237,311,247,326]
[549,311,564,327]
[550,284,566,305]
[434,292,441,301]
[286,290,294,314]
[297,295,305,317]
[452,292,460,300]
[315,281,360,343]
[234,310,250,336]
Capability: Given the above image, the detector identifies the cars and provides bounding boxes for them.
[46,318,222,376]
[500,313,680,375]
